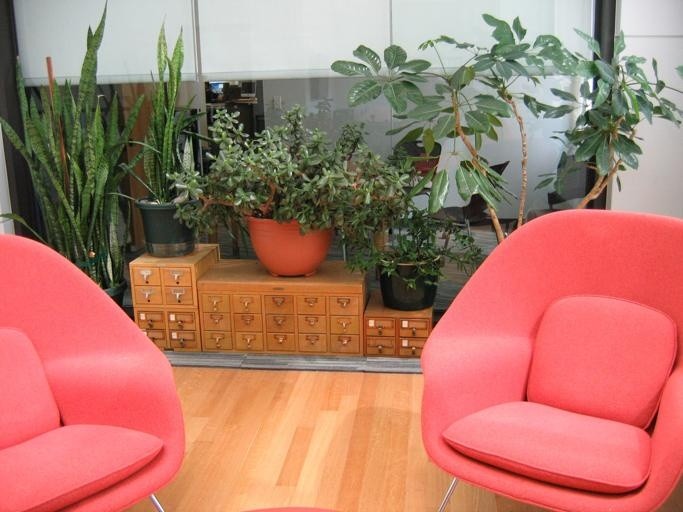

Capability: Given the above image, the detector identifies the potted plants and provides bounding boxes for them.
[0,0,141,309]
[165,105,416,277]
[372,206,480,312]
[105,20,209,257]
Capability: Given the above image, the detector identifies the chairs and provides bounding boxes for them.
[420,208,681,512]
[332,135,510,267]
[0,233,184,511]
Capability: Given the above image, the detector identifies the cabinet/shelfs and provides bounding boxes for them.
[196,258,369,360]
[127,243,218,353]
[362,288,436,358]
